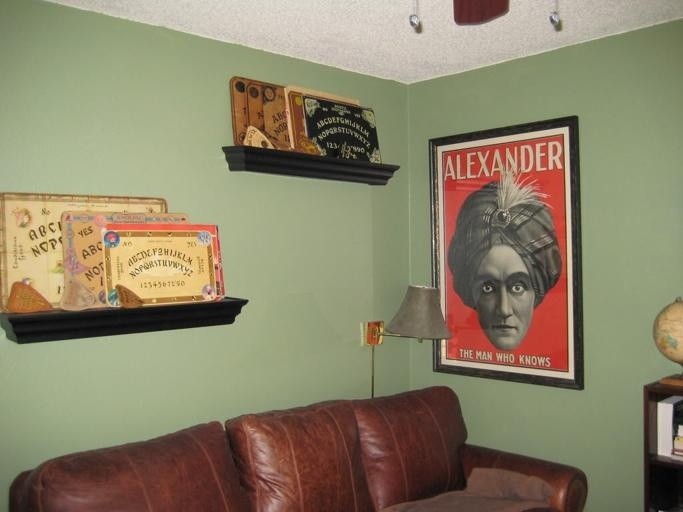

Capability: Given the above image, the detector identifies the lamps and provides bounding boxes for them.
[365,285,454,397]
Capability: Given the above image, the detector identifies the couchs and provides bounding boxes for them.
[13,386,588,512]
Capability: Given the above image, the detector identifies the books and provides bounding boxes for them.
[657,395,683,462]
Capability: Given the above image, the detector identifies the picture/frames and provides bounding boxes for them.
[427,112,584,392]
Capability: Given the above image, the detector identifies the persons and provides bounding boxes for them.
[447,179,561,351]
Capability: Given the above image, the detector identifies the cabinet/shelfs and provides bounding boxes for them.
[641,374,683,510]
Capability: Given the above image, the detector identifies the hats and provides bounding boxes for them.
[448,178,563,310]
